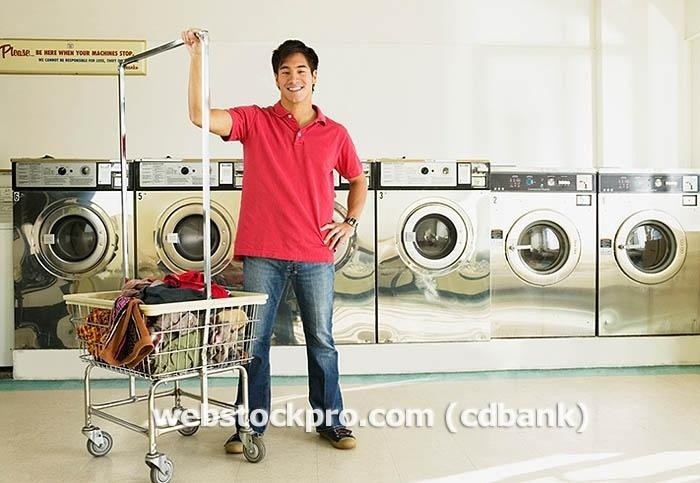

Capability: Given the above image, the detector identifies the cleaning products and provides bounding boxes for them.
[61,30,270,481]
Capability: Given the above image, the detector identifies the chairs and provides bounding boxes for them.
[224,433,245,454]
[320,425,358,450]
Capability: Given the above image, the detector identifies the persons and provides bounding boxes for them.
[181,20,368,455]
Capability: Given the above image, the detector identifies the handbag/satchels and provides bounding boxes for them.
[343,216,359,228]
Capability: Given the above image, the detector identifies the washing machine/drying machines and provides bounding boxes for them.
[12,157,699,349]
